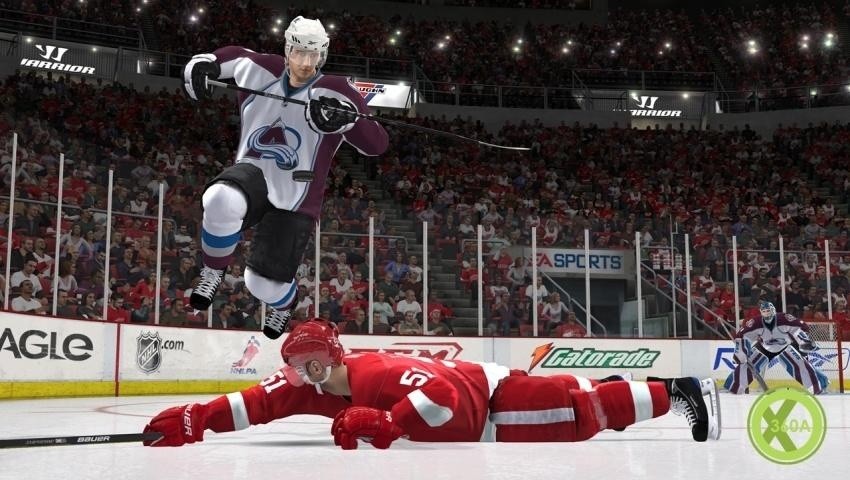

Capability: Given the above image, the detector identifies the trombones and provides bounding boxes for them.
[204,75,532,151]
